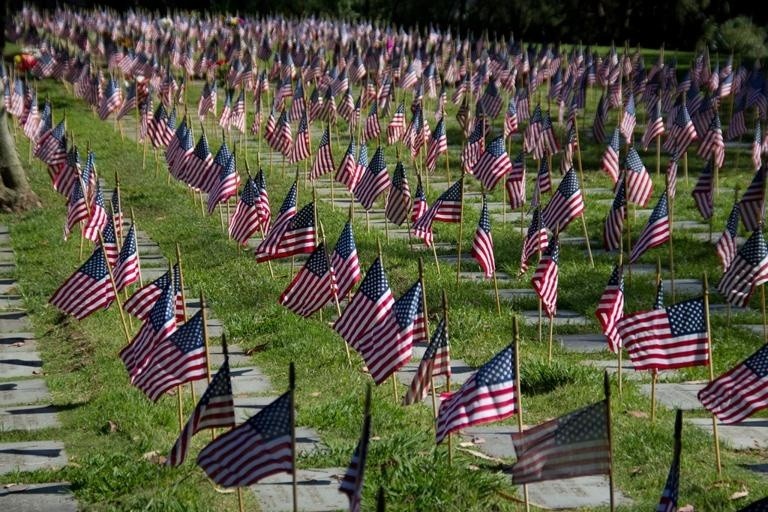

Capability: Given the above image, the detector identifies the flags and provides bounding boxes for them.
[3,6,768,511]
